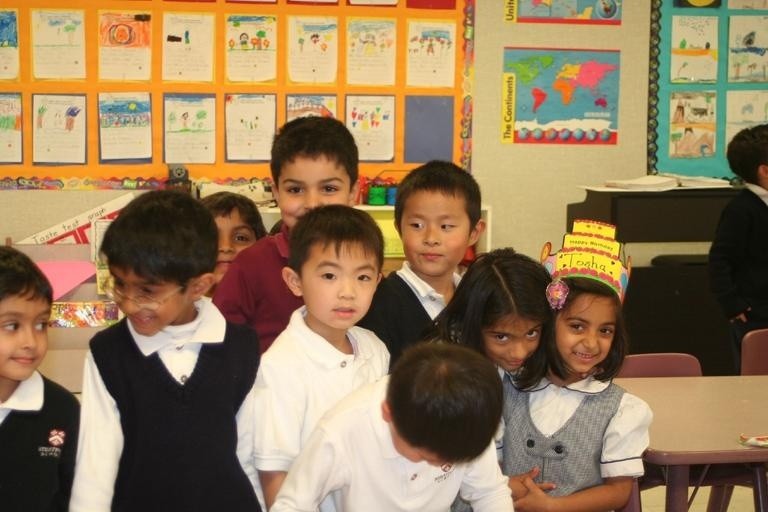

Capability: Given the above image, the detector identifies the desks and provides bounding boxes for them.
[608,376,768,512]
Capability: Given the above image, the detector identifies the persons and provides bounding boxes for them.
[0,244,81,511]
[273,340,514,511]
[454,279,654,511]
[709,126,768,374]
[420,245,570,393]
[211,114,357,354]
[68,188,261,512]
[237,203,396,512]
[355,160,485,369]
[198,191,268,285]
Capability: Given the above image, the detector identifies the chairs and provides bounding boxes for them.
[612,352,702,376]
[740,328,768,376]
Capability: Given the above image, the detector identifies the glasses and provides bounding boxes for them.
[101,279,185,309]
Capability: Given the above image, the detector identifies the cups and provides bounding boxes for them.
[367,186,397,205]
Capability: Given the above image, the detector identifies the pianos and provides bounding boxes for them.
[568,187,741,375]
